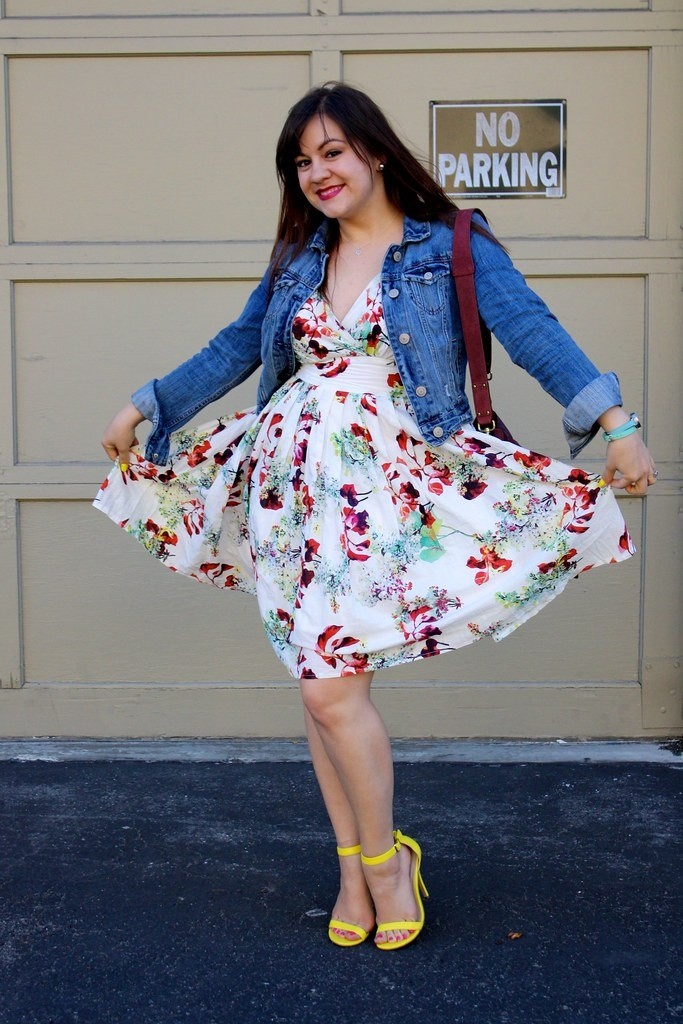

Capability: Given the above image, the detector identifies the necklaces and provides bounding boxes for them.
[340,205,400,257]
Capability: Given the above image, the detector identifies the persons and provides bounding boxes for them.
[103,81,661,954]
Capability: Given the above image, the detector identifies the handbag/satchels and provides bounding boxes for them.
[452,206,521,445]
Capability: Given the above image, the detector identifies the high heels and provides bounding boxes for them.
[330,831,430,951]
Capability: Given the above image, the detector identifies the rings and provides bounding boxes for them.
[653,470,659,477]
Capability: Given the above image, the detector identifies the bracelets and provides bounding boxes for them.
[602,413,641,441]
